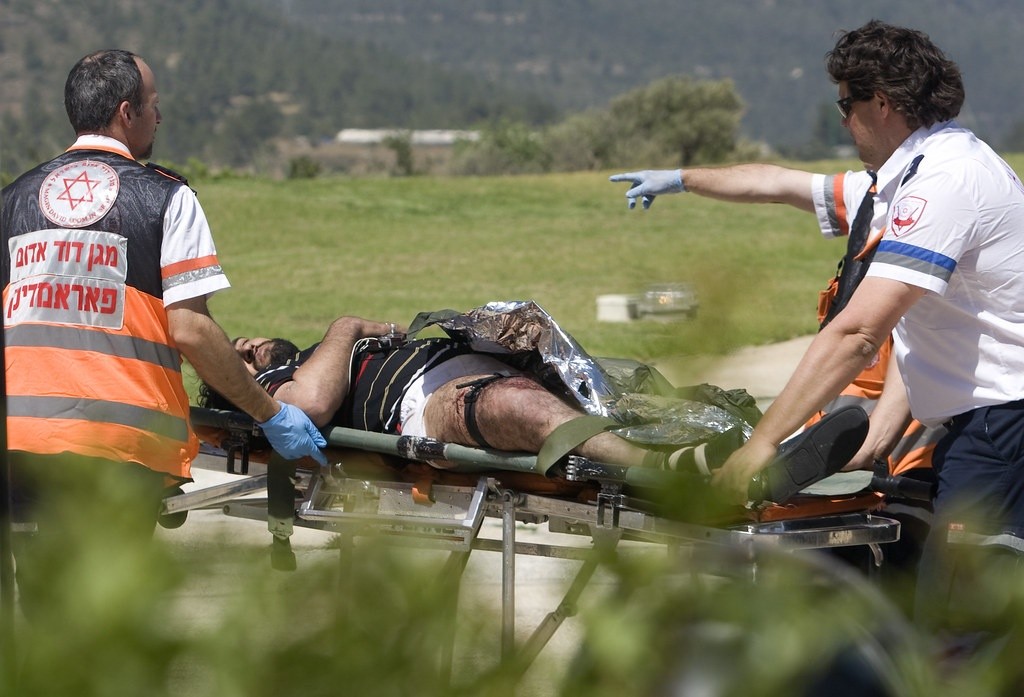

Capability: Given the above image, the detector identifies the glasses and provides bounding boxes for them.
[837,97,874,117]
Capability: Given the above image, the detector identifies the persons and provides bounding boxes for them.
[608,21,1024,697]
[0,49,329,697]
[195,314,870,510]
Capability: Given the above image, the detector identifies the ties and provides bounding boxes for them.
[816,171,878,332]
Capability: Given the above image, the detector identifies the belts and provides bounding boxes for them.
[942,400,1024,434]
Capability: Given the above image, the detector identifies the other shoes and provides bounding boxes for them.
[746,403,870,505]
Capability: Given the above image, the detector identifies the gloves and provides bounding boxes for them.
[257,401,327,468]
[610,169,688,209]
